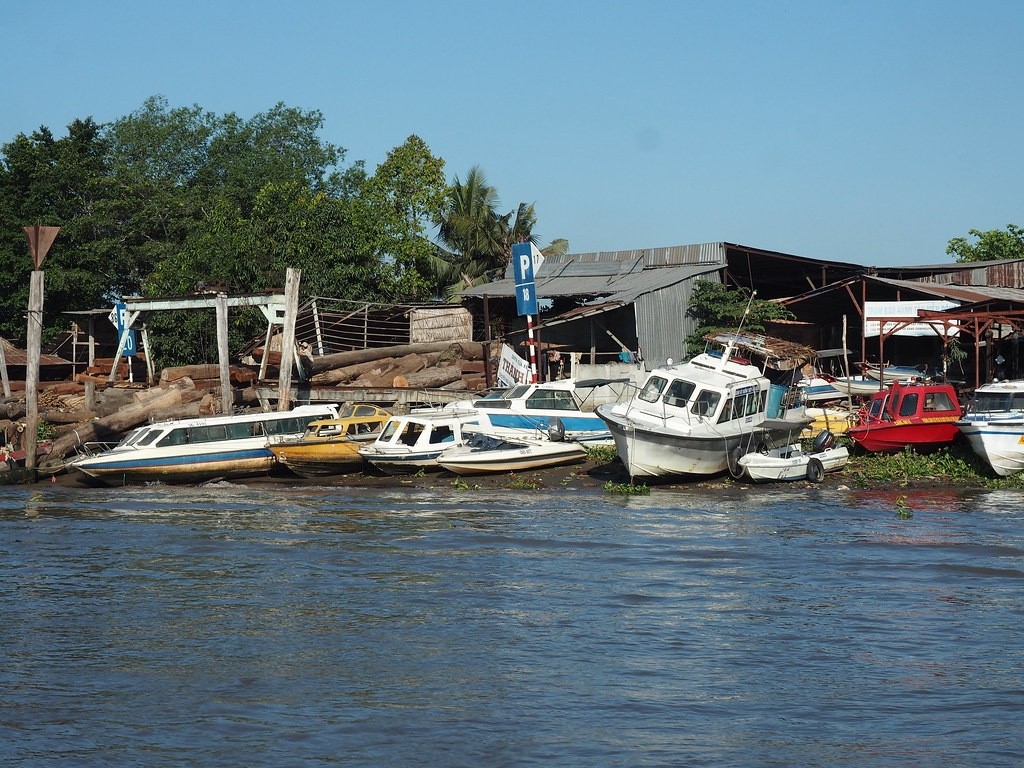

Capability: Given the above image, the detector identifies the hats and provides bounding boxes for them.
[301,342,308,347]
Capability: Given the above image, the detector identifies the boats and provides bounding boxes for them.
[73,404,339,483]
[815,361,944,395]
[435,432,589,475]
[410,378,637,447]
[954,377,1024,475]
[737,443,849,484]
[357,410,616,478]
[795,378,861,438]
[842,379,962,456]
[264,400,424,478]
[593,290,816,485]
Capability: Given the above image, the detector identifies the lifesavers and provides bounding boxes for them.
[807,458,825,483]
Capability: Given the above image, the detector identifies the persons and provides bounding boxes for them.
[549,353,558,381]
[300,342,314,373]
